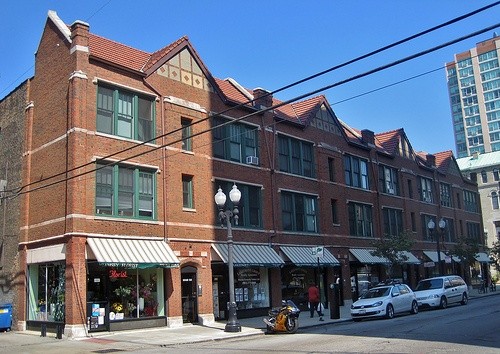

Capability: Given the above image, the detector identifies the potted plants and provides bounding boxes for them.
[127,302,136,318]
[119,269,127,282]
[109,269,119,282]
[129,283,153,311]
[113,283,134,317]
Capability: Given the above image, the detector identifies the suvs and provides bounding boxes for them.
[350,279,418,322]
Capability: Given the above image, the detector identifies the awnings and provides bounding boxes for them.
[87,238,180,268]
[210,244,285,268]
[349,249,494,264]
[279,247,340,266]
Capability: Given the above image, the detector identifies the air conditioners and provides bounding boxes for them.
[246,156,258,165]
[388,189,394,195]
[424,198,431,202]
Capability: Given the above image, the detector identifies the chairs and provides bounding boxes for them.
[182,306,190,323]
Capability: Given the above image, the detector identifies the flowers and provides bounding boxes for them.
[112,303,122,313]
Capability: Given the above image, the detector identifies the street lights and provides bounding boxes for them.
[427,217,445,276]
[215,183,242,332]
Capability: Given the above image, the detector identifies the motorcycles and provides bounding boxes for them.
[263,299,301,334]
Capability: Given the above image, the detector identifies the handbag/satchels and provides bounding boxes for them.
[317,303,324,312]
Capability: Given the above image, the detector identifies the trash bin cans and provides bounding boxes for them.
[0,303,13,332]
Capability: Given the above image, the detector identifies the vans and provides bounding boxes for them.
[413,276,468,309]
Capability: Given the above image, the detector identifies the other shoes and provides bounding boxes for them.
[310,315,313,318]
[319,313,324,317]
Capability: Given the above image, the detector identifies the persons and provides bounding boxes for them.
[480,277,495,293]
[308,282,324,318]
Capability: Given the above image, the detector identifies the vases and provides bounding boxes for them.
[115,313,124,320]
